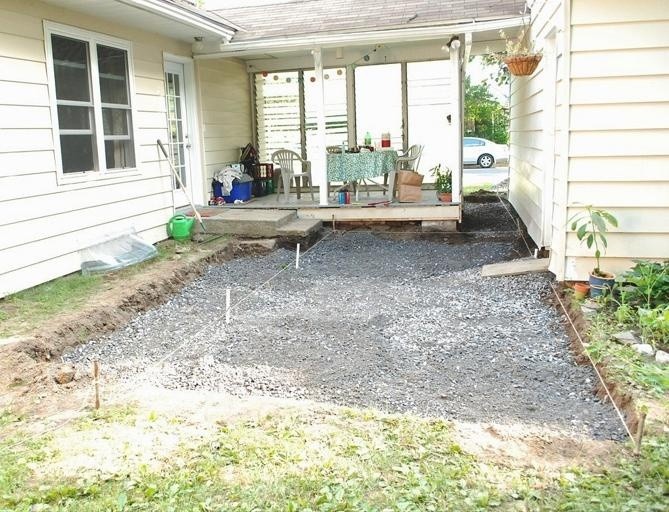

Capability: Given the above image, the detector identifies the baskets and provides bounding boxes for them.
[502,54,543,76]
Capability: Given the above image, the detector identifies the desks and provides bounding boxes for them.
[325,145,399,204]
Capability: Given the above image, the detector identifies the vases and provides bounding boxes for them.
[575,282,590,296]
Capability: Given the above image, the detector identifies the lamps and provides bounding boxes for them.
[334,47,344,60]
[440,34,461,54]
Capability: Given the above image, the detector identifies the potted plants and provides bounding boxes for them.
[567,201,620,303]
[431,164,451,201]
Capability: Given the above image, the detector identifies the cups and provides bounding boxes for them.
[381,134,390,147]
[351,147,358,152]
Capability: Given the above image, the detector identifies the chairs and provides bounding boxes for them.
[271,148,314,201]
[383,142,426,198]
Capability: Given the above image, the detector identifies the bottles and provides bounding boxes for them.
[364,131,371,147]
[338,190,350,204]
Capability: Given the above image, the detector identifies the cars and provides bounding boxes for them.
[462,136,509,168]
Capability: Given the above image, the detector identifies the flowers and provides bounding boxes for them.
[486,29,544,55]
